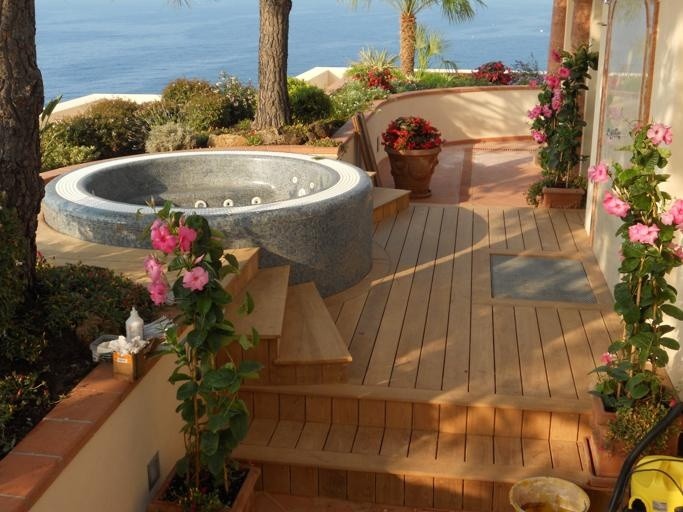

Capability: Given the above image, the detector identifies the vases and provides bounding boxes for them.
[146,455,261,512]
[541,173,585,210]
[385,144,442,200]
[583,384,683,488]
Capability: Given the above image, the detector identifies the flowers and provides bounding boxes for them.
[524,41,599,209]
[587,105,683,460]
[138,196,258,512]
[380,117,444,151]
